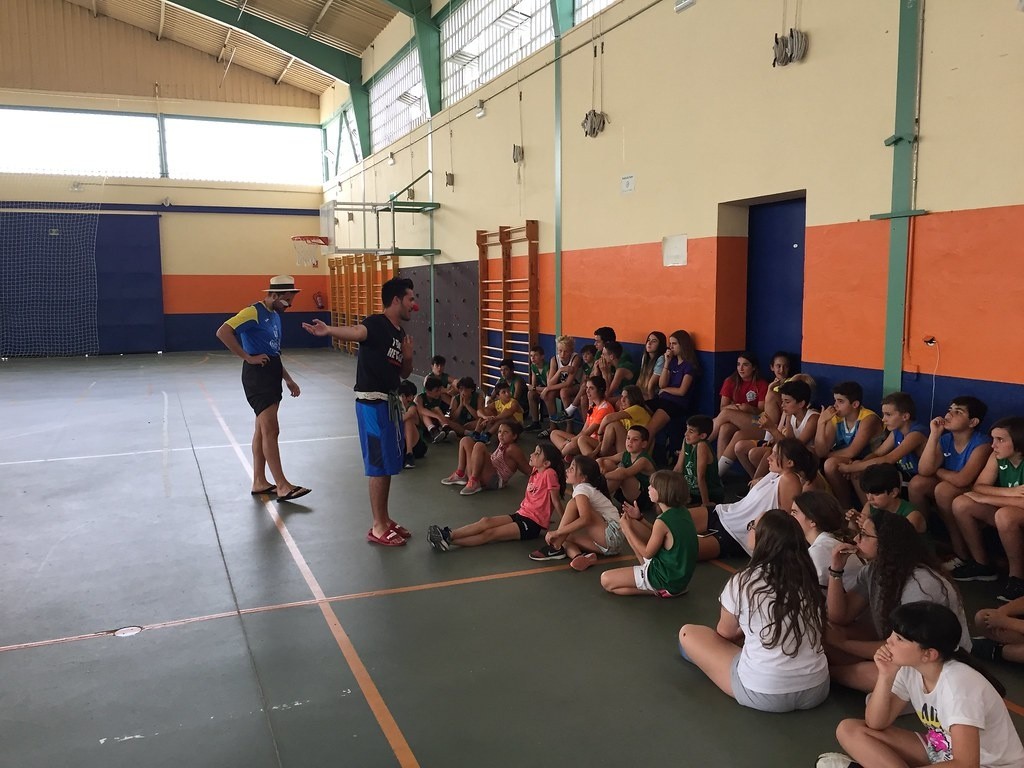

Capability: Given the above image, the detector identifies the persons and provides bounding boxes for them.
[816,599,1024,768]
[427,439,564,554]
[600,470,699,597]
[440,421,533,495]
[678,509,830,713]
[400,324,1024,693]
[216,276,312,502]
[303,278,418,546]
[529,455,625,571]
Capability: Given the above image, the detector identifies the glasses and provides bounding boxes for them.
[859,528,879,538]
[747,520,756,531]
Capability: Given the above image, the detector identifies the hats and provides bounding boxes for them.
[261,276,301,292]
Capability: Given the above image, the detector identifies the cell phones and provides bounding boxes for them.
[840,549,858,554]
[697,529,719,537]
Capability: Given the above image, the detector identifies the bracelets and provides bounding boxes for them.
[597,432,604,436]
[533,387,536,391]
[636,513,644,521]
[828,567,845,578]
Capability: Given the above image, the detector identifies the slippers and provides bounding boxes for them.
[368,522,412,538]
[276,486,312,502]
[368,533,407,546]
[252,485,276,495]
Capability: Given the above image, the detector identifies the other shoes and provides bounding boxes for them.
[537,429,551,439]
[404,453,416,468]
[815,753,864,768]
[446,430,456,442]
[472,433,479,441]
[659,589,673,598]
[524,423,543,432]
[565,455,574,463]
[478,434,492,445]
[427,525,451,552]
[434,432,446,444]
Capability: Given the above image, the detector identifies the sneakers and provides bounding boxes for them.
[549,409,574,423]
[460,478,482,495]
[529,543,566,561]
[570,552,597,571]
[935,554,1024,600]
[441,472,467,485]
